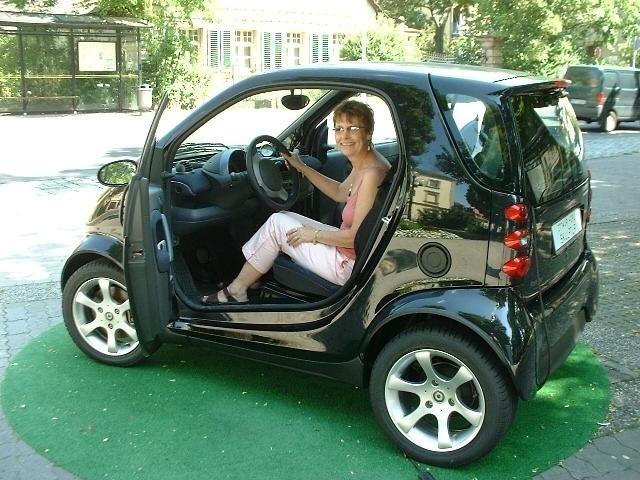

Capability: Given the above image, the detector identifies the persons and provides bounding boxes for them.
[198,98,392,307]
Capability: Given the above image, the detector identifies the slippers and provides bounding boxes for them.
[200,286,252,306]
[216,278,261,296]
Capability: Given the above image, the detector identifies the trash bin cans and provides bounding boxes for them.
[137,83,154,116]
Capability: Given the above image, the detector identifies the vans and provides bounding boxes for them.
[562,65,640,131]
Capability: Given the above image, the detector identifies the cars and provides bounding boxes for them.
[57,59,602,469]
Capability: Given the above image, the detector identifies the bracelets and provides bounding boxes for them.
[298,164,307,173]
[313,229,322,245]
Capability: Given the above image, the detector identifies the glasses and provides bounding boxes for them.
[332,125,366,136]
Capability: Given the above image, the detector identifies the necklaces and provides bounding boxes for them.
[347,153,370,198]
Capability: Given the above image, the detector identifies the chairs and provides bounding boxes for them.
[267,154,400,307]
